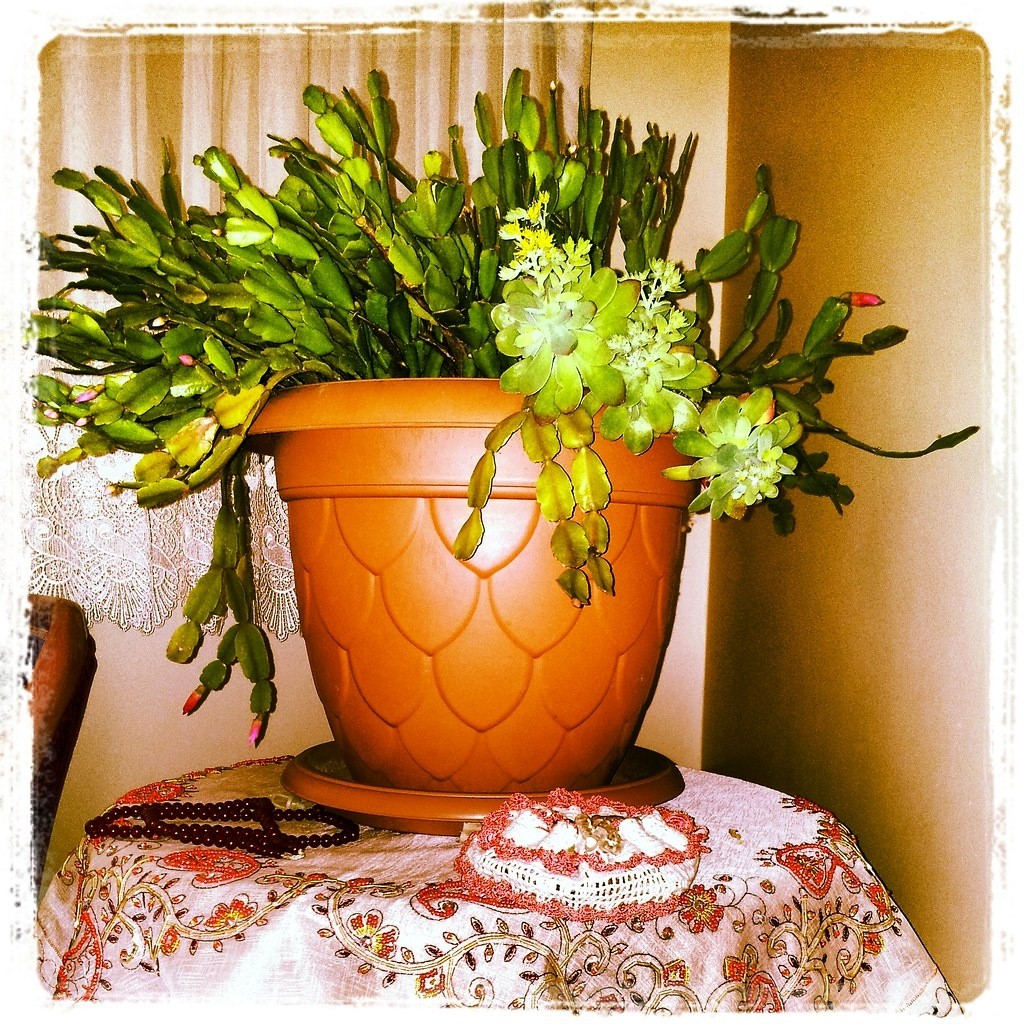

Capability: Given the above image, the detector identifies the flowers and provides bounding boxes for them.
[32,44,979,753]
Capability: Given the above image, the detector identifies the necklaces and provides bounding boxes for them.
[84,795,361,862]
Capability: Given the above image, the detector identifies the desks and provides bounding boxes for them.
[32,762,957,1022]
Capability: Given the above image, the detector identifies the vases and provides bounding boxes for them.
[246,381,700,794]
